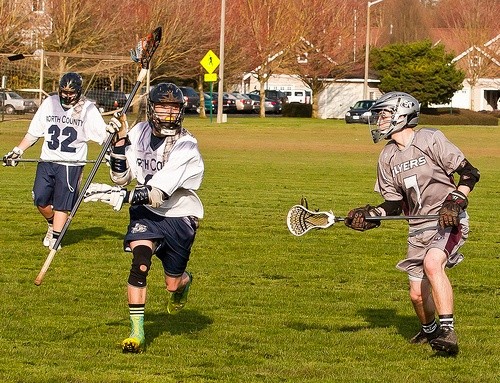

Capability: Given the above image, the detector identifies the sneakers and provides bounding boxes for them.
[42,222,54,246]
[432,329,458,354]
[410,325,440,344]
[49,238,63,251]
[121,334,146,355]
[167,270,193,315]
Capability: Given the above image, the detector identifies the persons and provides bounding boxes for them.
[3,73,111,251]
[343,91,481,355]
[83,83,205,351]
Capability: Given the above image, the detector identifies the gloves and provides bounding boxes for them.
[105,112,130,155]
[438,191,468,235]
[345,204,382,230]
[83,187,128,211]
[2,146,23,167]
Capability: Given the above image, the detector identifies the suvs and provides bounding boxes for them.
[126,86,200,113]
[85,90,126,114]
[250,90,288,106]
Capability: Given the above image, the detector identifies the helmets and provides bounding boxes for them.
[146,84,185,138]
[367,91,420,143]
[59,73,83,111]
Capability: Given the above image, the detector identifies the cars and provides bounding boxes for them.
[345,100,378,124]
[200,92,260,113]
[0,89,35,114]
[244,94,279,113]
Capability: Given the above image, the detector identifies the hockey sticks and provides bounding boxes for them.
[286,196,440,236]
[33,27,163,286]
[0,159,109,164]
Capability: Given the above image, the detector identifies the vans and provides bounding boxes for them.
[278,90,306,105]
[15,89,52,105]
